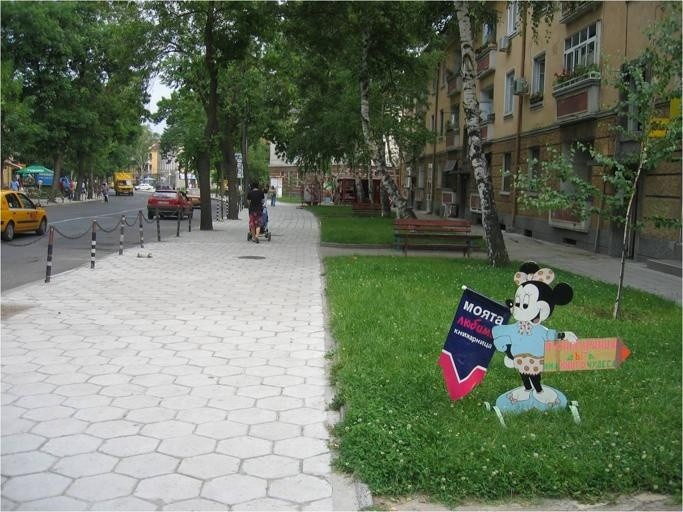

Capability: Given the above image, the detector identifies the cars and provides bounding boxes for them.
[0,190,47,241]
[134,183,154,190]
[147,188,200,220]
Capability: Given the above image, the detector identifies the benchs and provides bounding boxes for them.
[392,217,483,259]
[351,202,382,217]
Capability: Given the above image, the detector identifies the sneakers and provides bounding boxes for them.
[251,235,259,243]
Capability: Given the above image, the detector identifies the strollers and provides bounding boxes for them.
[247,207,271,241]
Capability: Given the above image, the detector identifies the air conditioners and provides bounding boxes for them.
[499,37,511,52]
[513,79,528,95]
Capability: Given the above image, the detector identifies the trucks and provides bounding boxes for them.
[113,172,133,196]
[35,174,70,193]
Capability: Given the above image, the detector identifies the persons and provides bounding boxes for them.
[69,179,75,199]
[100,181,108,204]
[263,183,277,207]
[37,178,44,190]
[81,181,87,201]
[9,175,20,192]
[244,181,265,242]
